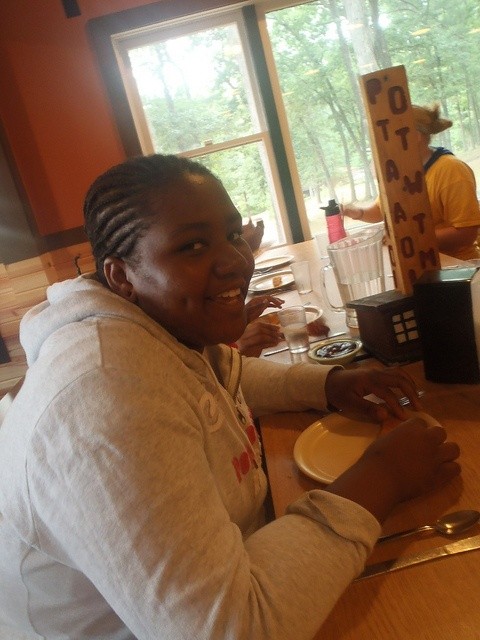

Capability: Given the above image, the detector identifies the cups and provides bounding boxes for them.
[290,259,314,294]
[319,224,386,330]
[277,305,311,354]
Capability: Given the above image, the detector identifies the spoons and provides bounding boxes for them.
[374,509,480,544]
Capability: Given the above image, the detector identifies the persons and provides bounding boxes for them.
[337,104,480,261]
[0,154,462,634]
[233,294,285,358]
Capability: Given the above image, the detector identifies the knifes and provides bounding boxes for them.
[263,331,347,357]
[350,532,479,584]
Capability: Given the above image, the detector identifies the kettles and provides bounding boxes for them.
[320,199,347,245]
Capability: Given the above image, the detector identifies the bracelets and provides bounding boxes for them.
[352,207,363,220]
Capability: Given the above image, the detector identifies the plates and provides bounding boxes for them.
[255,255,295,270]
[277,304,324,335]
[247,269,295,295]
[292,401,443,486]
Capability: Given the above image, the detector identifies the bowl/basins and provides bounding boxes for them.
[307,337,363,365]
